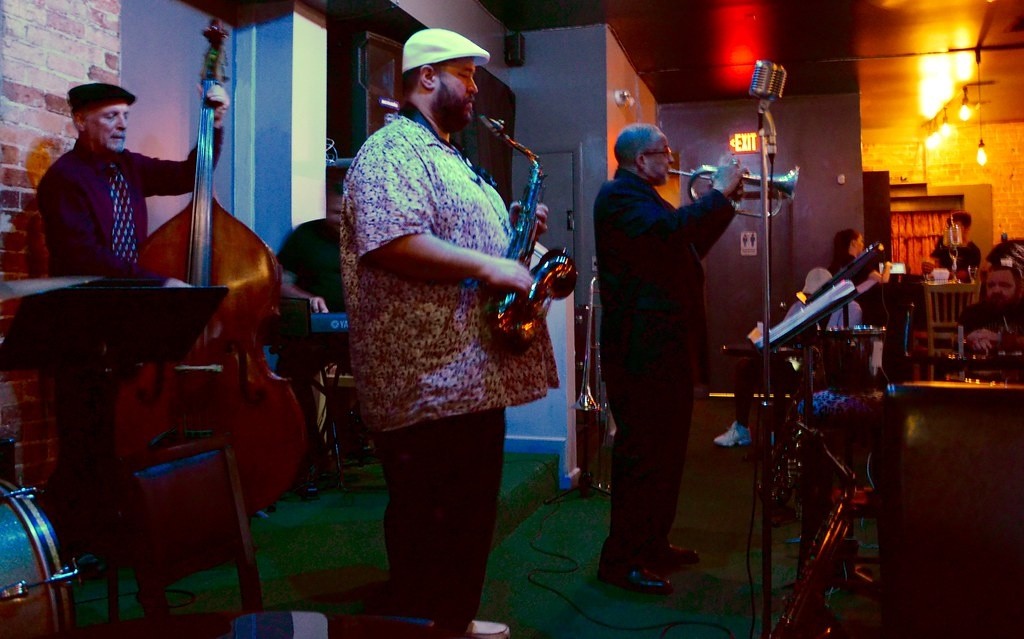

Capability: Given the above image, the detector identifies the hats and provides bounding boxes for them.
[402,28,490,73]
[69,83,135,113]
[802,268,833,295]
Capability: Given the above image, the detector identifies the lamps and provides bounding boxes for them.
[614,89,635,108]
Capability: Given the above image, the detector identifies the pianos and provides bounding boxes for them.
[279,312,349,492]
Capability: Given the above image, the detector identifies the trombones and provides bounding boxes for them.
[573,277,613,494]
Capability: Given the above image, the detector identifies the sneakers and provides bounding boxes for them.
[714,421,751,448]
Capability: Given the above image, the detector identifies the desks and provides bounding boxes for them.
[38,611,473,639]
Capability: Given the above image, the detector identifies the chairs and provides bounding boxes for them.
[49,336,262,614]
[910,280,981,381]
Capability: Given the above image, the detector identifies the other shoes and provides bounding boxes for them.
[465,621,510,639]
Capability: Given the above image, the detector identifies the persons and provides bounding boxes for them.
[921,211,980,323]
[277,180,378,498]
[828,228,893,326]
[593,124,751,596]
[713,267,864,448]
[338,28,550,639]
[36,83,230,578]
[956,264,1024,381]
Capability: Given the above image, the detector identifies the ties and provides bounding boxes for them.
[109,170,137,267]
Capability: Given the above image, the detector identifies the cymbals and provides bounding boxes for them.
[3,278,100,298]
[1,278,99,299]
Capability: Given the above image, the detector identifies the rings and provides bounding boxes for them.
[731,161,739,168]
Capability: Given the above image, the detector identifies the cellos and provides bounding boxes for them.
[102,17,307,528]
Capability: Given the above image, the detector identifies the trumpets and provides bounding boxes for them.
[667,169,800,217]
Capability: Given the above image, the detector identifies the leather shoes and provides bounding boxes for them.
[598,563,673,595]
[644,542,699,567]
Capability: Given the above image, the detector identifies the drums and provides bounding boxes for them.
[1,481,78,639]
[0,479,79,639]
[821,325,887,391]
[874,377,1024,639]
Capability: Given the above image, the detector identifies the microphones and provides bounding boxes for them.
[749,59,787,165]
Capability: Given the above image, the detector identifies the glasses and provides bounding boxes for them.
[642,145,671,158]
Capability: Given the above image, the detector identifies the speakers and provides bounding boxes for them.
[505,31,526,66]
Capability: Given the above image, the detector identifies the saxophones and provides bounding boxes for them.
[480,114,570,354]
[771,423,857,638]
[479,114,576,354]
